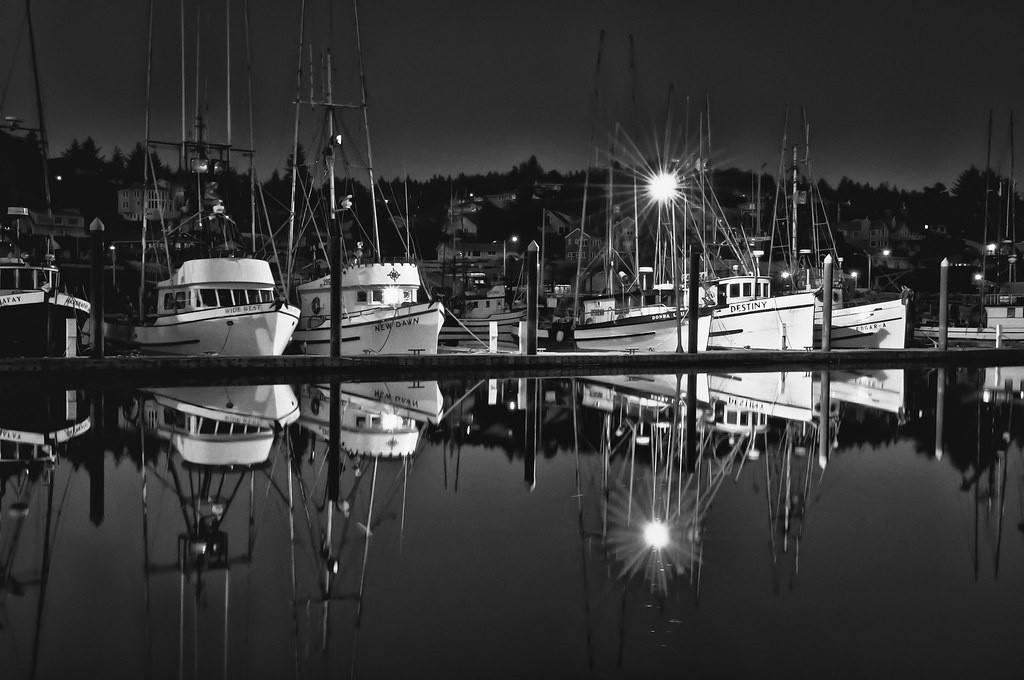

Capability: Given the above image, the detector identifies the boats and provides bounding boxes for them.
[0,359,929,680]
[0,0,918,356]
